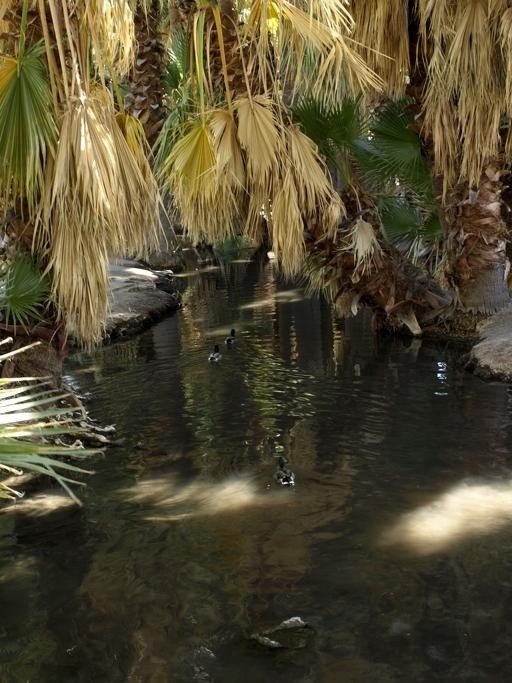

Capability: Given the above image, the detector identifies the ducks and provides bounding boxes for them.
[208,344,222,362]
[224,329,237,345]
[273,457,295,486]
